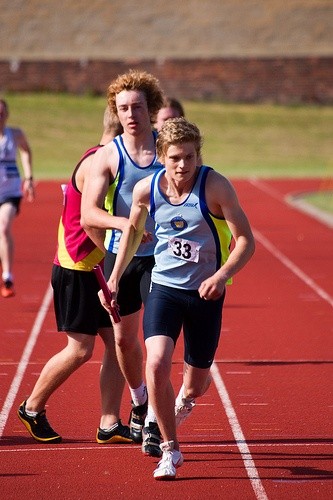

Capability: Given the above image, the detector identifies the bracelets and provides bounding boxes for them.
[25,177,32,180]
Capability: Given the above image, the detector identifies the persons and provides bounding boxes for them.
[98,121,255,477]
[155,98,185,131]
[0,100,36,298]
[84,71,166,454]
[17,105,133,443]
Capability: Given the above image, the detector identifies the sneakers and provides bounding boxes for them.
[174,383,196,428]
[17,399,63,444]
[152,440,183,480]
[128,392,148,444]
[0,278,15,297]
[141,422,164,458]
[96,418,133,443]
